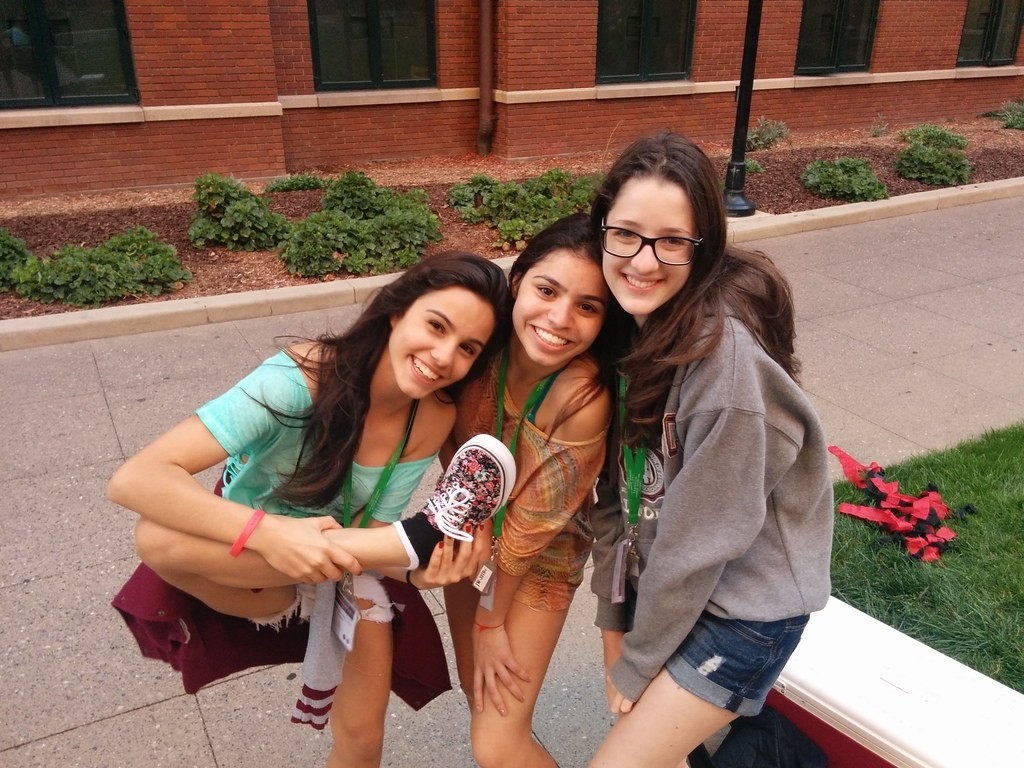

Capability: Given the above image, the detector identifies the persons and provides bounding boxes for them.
[587,133,835,768]
[109,251,513,768]
[440,212,615,768]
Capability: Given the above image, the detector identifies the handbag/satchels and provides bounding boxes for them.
[689,704,827,768]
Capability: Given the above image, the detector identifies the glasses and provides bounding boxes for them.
[600,214,704,265]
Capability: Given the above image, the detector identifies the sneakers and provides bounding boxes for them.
[421,433,516,544]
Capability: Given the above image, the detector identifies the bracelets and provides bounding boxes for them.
[474,621,504,632]
[406,570,421,591]
[229,508,266,558]
